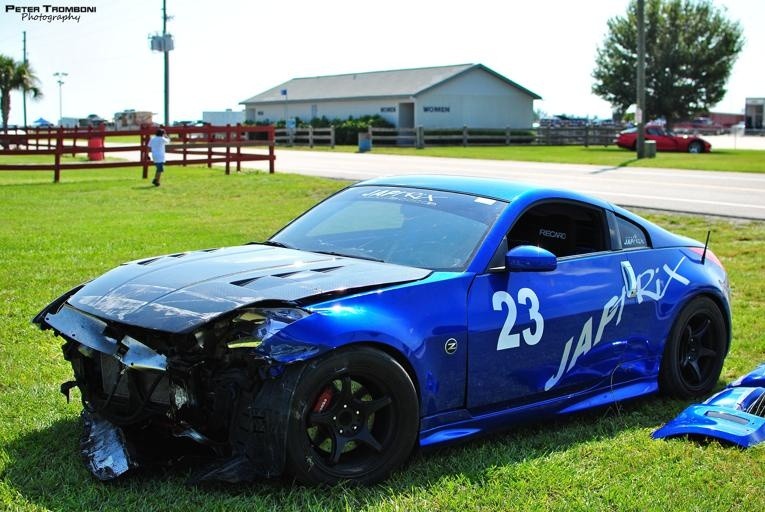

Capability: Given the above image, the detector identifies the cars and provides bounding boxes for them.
[672,117,724,135]
[613,120,711,153]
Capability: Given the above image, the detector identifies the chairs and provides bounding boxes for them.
[385,216,606,270]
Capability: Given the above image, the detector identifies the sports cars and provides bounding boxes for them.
[30,174,732,492]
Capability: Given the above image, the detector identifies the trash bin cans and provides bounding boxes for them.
[358,133,372,153]
[87,137,103,161]
[643,140,656,158]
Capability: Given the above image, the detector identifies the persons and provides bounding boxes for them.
[286,115,296,146]
[147,130,170,187]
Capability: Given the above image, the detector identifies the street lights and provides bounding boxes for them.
[53,71,69,125]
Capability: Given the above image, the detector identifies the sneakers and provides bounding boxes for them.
[152,178,162,188]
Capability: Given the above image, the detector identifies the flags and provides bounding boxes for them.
[280,88,288,96]
